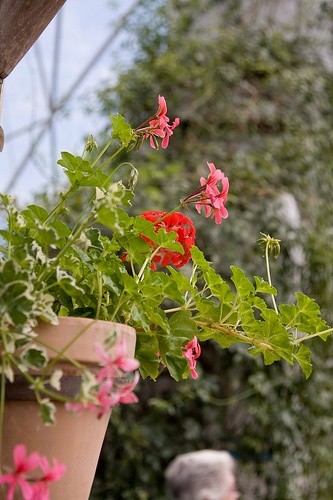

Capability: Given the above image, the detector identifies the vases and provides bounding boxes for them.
[0,315,139,499]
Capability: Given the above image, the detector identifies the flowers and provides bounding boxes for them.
[0,97,232,500]
